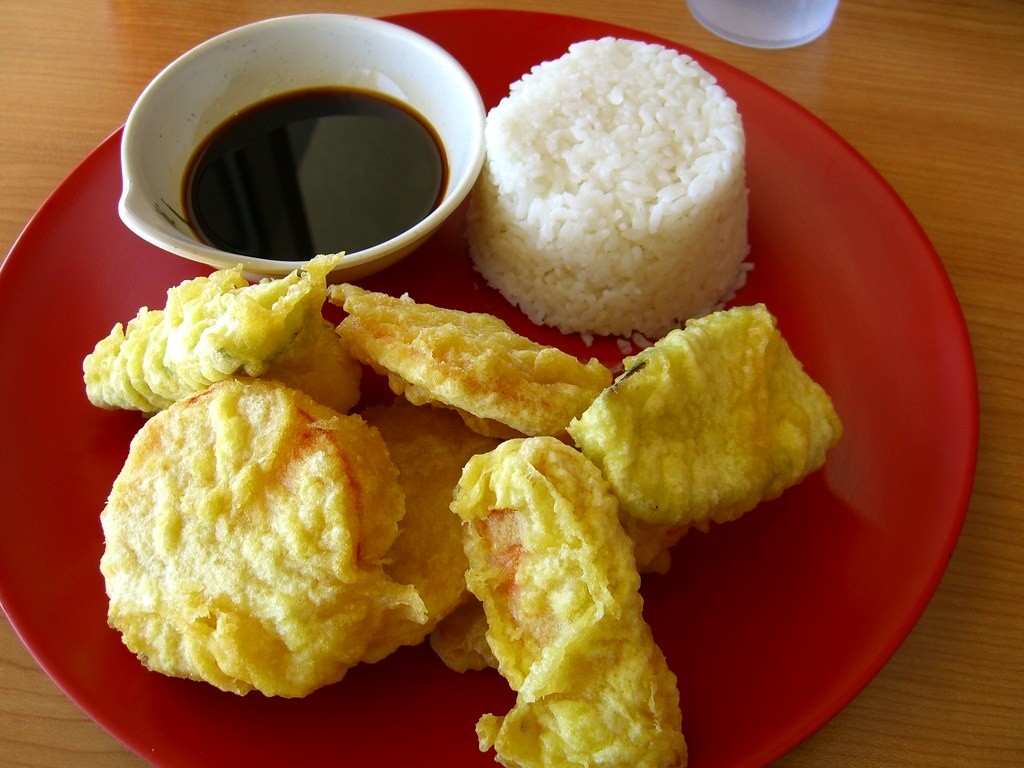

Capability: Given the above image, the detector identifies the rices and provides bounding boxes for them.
[464,36,755,353]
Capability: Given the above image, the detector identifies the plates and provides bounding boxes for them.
[0,7,979,766]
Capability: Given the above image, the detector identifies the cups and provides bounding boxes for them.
[685,0,838,50]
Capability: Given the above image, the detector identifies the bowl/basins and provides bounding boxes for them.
[117,14,487,283]
